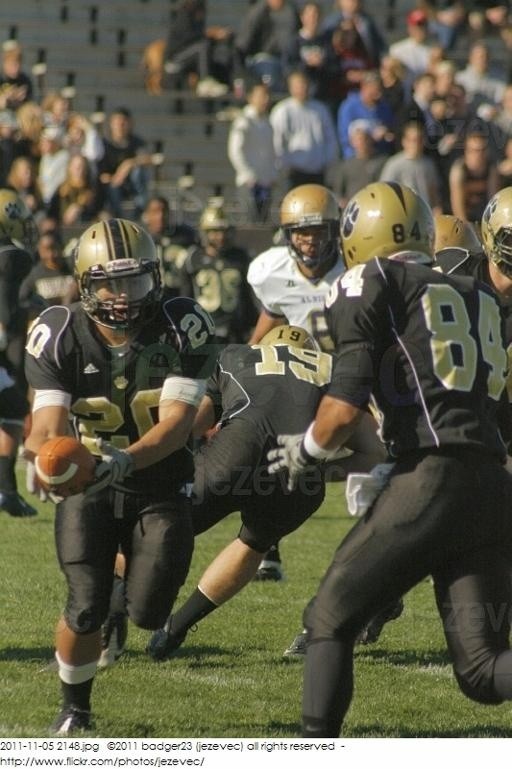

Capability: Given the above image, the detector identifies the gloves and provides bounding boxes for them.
[266,434,326,493]
[18,447,65,505]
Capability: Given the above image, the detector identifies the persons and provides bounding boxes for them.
[0,1,512,737]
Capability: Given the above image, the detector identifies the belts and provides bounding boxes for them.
[84,442,133,496]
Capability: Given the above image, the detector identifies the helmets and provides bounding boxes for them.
[199,204,235,229]
[340,181,436,268]
[481,185,512,280]
[1,189,40,255]
[432,215,485,278]
[73,219,162,330]
[281,184,341,268]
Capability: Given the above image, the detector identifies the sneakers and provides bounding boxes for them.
[97,573,127,668]
[356,596,403,645]
[146,614,186,661]
[280,629,311,662]
[51,703,92,738]
[0,491,38,517]
[252,540,282,580]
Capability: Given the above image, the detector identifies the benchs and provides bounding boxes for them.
[0,0,512,257]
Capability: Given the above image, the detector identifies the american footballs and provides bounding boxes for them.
[35,438,94,497]
